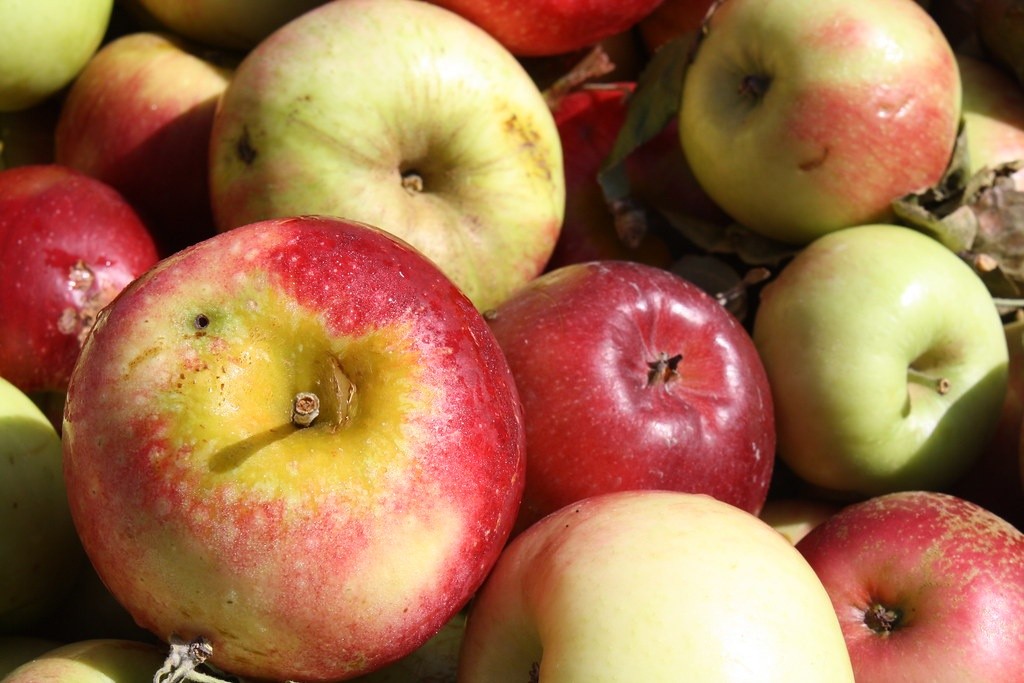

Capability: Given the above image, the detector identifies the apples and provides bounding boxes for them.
[0,1,1024,682]
